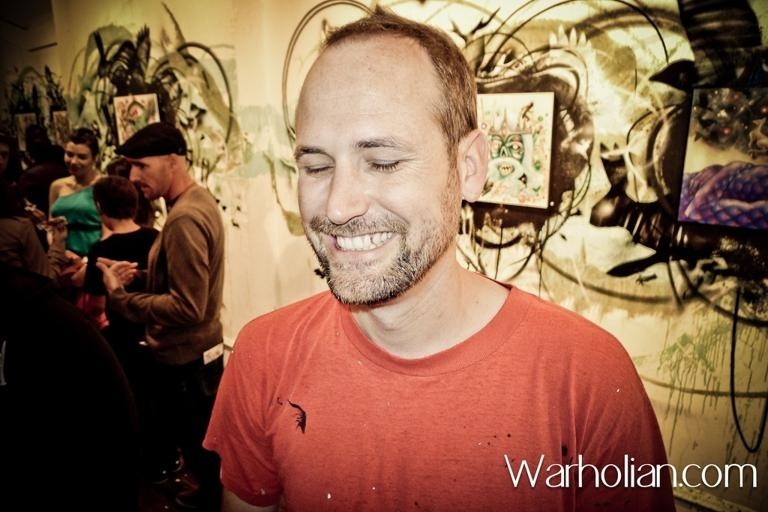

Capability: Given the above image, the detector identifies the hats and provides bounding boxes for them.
[115,123,186,159]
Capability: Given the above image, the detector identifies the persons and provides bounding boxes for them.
[201,11,677,510]
[0,122,226,510]
